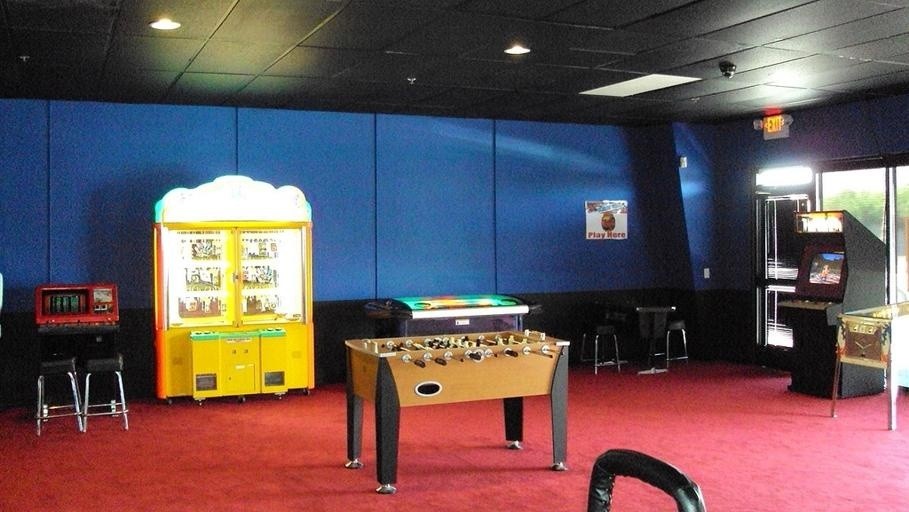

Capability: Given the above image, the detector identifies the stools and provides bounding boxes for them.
[665,319,690,369]
[34,351,85,437]
[579,323,623,377]
[81,346,130,436]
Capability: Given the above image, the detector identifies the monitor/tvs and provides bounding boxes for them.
[808,252,845,286]
[393,294,524,311]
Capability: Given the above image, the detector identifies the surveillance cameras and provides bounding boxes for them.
[719,63,737,78]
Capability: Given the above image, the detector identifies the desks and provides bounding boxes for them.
[596,298,677,377]
